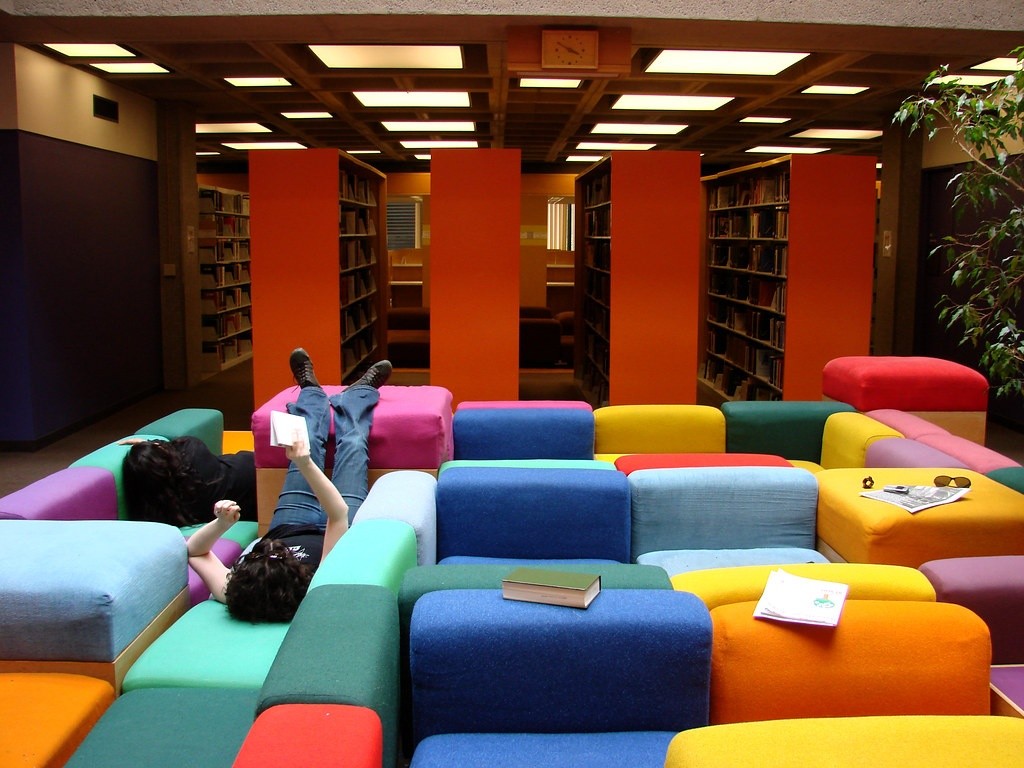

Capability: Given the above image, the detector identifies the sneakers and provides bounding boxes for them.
[341,360,392,391]
[290,348,322,393]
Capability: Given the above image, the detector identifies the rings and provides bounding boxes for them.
[215,510,220,513]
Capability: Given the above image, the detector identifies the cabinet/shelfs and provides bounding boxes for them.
[695,154,790,408]
[573,149,613,410]
[193,186,252,374]
[337,147,391,390]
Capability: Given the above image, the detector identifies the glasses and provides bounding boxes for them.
[934,476,971,488]
[244,552,286,560]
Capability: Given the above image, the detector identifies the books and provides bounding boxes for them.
[269,411,310,451]
[581,169,613,407]
[339,167,386,388]
[199,188,254,370]
[502,566,602,610]
[705,168,789,400]
[753,569,848,626]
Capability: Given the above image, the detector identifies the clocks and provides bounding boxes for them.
[541,29,599,69]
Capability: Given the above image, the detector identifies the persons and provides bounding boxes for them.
[187,348,391,624]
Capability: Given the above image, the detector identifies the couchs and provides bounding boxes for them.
[386,303,561,370]
